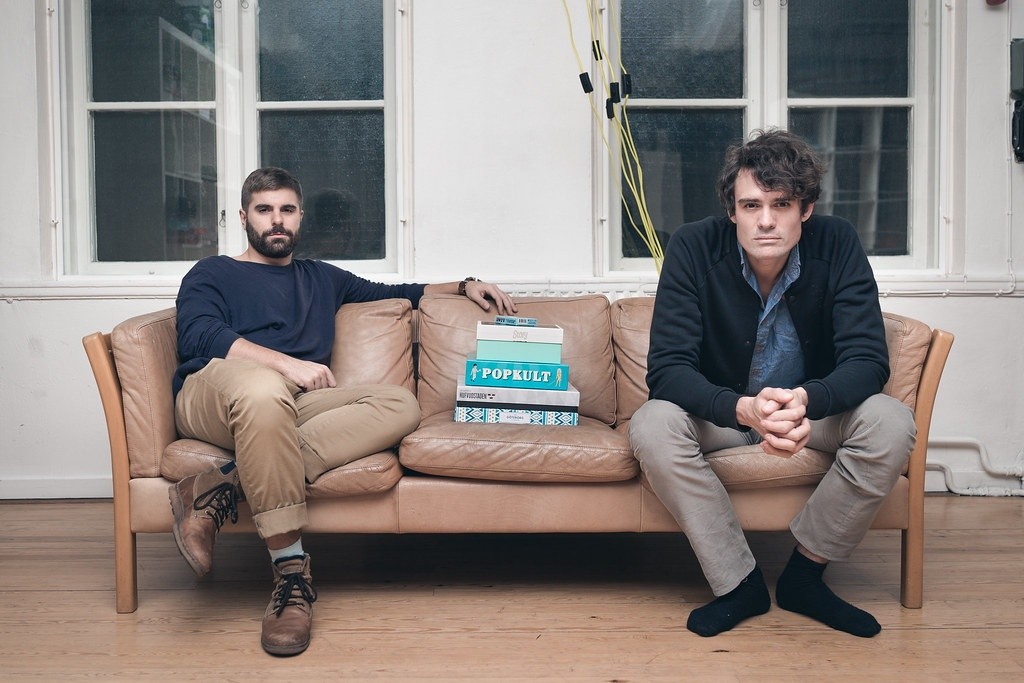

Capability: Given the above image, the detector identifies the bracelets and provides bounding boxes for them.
[458,277,482,296]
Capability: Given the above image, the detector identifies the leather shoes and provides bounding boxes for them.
[260,553,318,656]
[168,460,246,577]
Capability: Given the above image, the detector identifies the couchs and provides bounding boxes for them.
[82,294,955,615]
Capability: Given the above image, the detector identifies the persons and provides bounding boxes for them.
[168,167,519,658]
[626,129,919,638]
